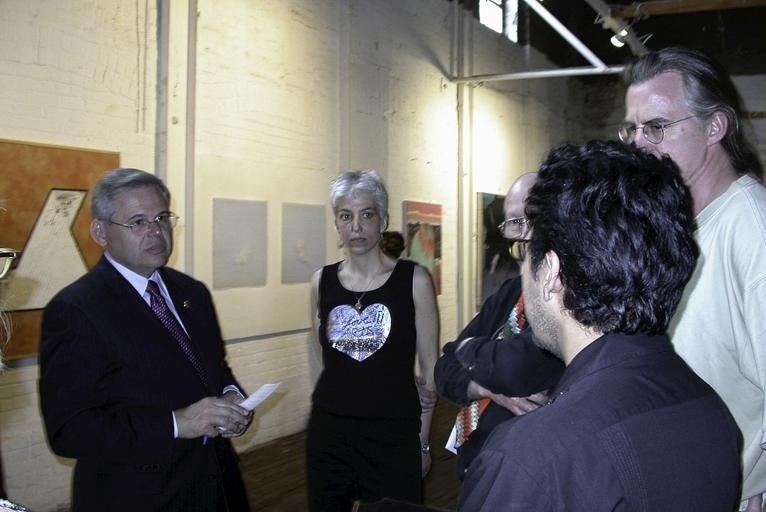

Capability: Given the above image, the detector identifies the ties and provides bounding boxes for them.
[144,279,209,395]
[454,289,526,452]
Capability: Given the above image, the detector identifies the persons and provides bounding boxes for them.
[459,139,745,512]
[303,170,441,511]
[36,168,255,512]
[616,44,766,512]
[431,172,567,481]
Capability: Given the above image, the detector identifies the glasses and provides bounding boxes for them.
[496,216,532,239]
[618,111,705,146]
[97,211,181,237]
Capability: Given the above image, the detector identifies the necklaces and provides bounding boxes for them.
[345,267,381,309]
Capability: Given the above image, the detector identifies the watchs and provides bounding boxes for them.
[421,444,430,454]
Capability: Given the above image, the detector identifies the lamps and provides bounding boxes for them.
[609,11,650,49]
